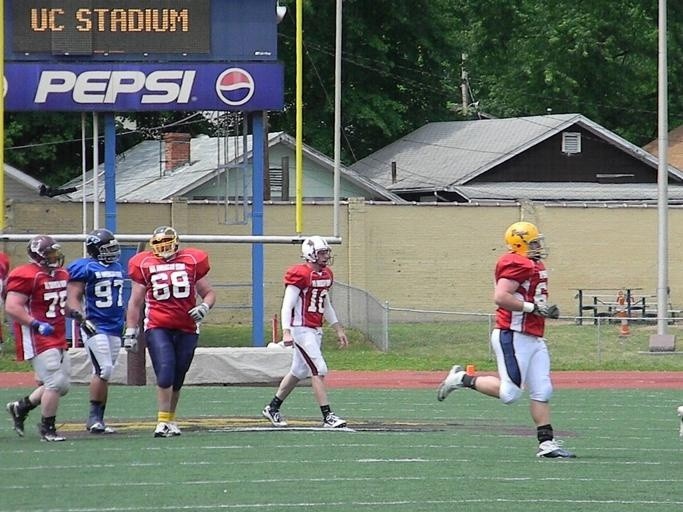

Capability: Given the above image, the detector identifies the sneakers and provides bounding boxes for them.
[6,402,25,437]
[154,424,180,436]
[87,419,114,434]
[40,423,66,442]
[537,440,577,459]
[437,363,467,401]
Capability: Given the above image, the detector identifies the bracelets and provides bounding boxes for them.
[522,302,534,313]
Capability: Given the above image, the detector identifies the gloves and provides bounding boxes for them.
[548,304,559,319]
[533,302,548,318]
[188,303,211,322]
[122,327,139,354]
[71,308,98,336]
[30,319,54,336]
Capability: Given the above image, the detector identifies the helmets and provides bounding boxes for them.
[26,235,65,269]
[505,222,549,260]
[302,235,334,265]
[150,226,178,260]
[85,228,120,263]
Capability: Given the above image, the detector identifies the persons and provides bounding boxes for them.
[435,221,577,459]
[64,228,140,435]
[2,235,71,442]
[121,226,217,439]
[260,235,350,429]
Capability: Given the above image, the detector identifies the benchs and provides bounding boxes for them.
[570,287,676,325]
